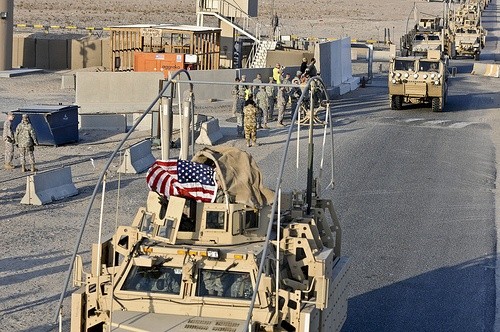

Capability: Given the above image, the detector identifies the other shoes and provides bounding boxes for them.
[31,169,38,172]
[22,168,27,172]
[4,164,15,169]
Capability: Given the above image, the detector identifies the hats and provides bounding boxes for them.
[260,86,266,89]
[8,112,15,116]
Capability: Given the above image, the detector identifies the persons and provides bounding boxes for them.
[136,267,255,300]
[232,57,321,148]
[15,114,39,172]
[270,11,279,36]
[3,111,16,169]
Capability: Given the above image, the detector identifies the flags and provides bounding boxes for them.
[146,159,218,203]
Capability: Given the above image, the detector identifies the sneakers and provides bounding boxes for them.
[263,126,270,129]
[247,143,251,147]
[252,142,260,147]
[277,122,284,127]
[257,125,263,129]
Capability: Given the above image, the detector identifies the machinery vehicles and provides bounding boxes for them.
[400,19,456,58]
[452,0,491,27]
[388,51,449,112]
[56,69,352,332]
[455,26,487,60]
